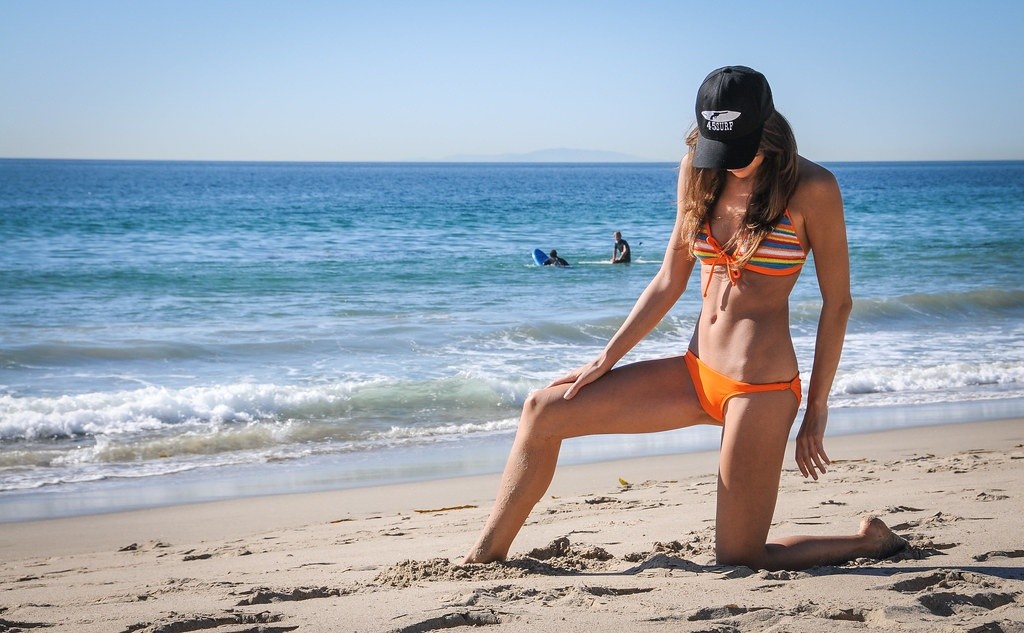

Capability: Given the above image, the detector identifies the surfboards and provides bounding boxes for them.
[532,248,549,267]
[578,260,663,264]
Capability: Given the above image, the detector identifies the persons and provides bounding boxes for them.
[611,231,631,262]
[464,65,911,572]
[543,250,569,265]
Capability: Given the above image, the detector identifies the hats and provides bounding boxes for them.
[692,67,774,170]
